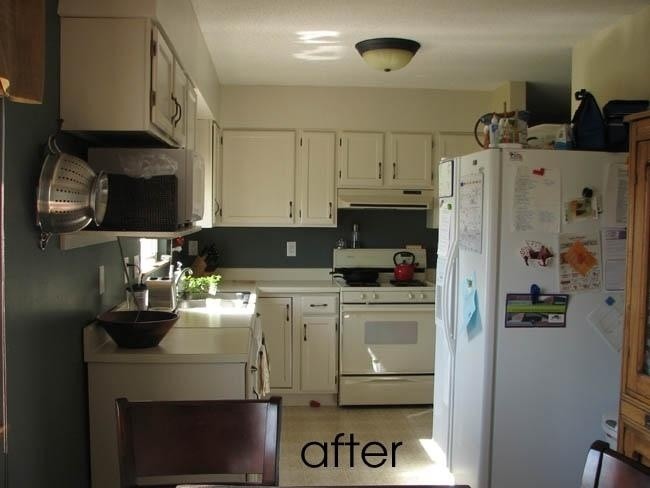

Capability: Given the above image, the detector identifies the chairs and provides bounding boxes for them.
[582,441,648,485]
[112,395,283,488]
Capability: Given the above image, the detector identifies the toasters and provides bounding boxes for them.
[146,276,177,310]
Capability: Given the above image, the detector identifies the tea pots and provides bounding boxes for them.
[392,251,419,279]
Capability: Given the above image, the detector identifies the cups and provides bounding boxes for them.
[474,110,501,149]
[126,283,148,310]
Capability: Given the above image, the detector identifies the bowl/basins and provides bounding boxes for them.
[95,310,179,348]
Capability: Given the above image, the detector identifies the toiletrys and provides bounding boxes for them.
[174,261,186,297]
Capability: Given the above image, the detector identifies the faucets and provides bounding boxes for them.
[168,263,194,286]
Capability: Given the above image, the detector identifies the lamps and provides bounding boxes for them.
[354,37,420,72]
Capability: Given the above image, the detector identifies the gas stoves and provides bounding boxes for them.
[333,275,435,305]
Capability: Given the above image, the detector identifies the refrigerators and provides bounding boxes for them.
[433,146,626,488]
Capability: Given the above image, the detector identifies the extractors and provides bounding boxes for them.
[345,186,430,209]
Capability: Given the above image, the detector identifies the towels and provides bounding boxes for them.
[255,345,270,400]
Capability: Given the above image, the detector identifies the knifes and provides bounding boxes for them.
[201,241,220,272]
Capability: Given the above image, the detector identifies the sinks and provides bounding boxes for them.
[180,291,246,300]
[178,299,245,312]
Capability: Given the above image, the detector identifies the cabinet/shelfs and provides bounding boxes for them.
[87,310,262,488]
[302,131,336,224]
[256,295,338,392]
[341,130,433,187]
[193,119,221,230]
[224,130,296,225]
[59,17,187,148]
[426,132,488,229]
[616,107,649,463]
[187,85,197,148]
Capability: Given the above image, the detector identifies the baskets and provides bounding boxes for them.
[34,134,108,251]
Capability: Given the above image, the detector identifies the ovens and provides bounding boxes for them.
[338,304,433,406]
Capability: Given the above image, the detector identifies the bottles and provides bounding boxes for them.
[351,223,362,249]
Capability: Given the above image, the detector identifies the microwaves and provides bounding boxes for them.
[85,147,207,229]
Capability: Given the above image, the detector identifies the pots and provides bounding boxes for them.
[328,268,383,281]
